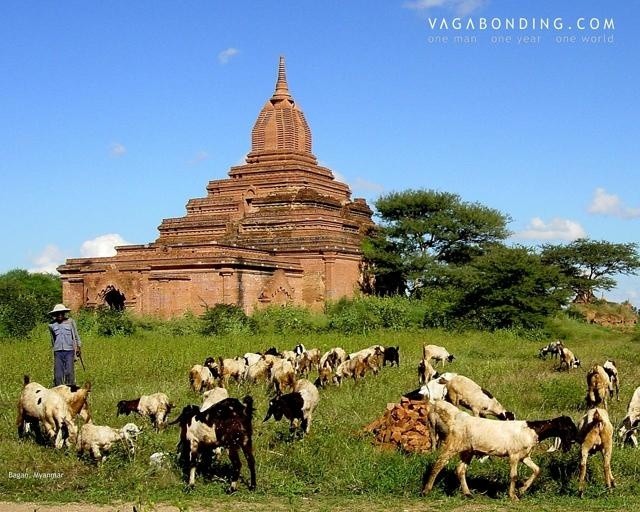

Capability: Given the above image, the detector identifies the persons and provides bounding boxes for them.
[49,304,82,386]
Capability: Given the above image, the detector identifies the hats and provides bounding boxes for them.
[47,302,72,316]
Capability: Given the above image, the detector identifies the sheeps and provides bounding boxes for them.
[169,342,401,492]
[413,339,640,503]
[17,374,174,465]
